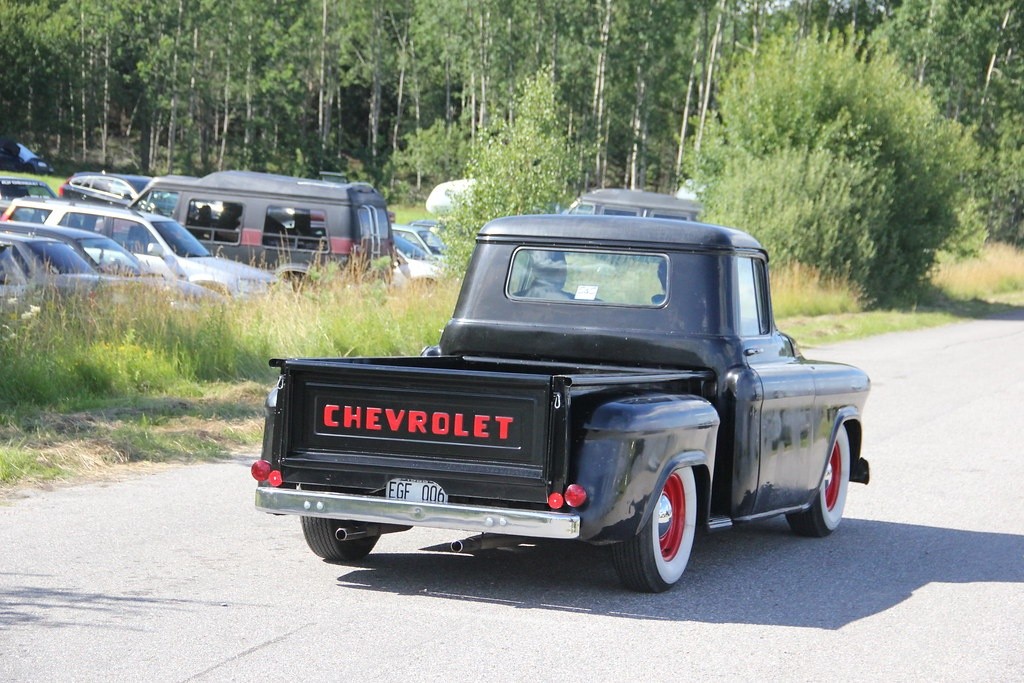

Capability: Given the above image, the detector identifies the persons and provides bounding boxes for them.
[651,257,667,305]
[512,249,576,300]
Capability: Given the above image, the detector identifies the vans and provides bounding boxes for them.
[118,168,393,296]
[560,187,704,222]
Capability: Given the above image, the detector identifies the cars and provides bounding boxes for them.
[388,217,452,287]
[0,176,54,218]
[0,220,226,312]
[1,233,133,309]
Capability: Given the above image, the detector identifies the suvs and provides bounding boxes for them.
[0,196,283,304]
[56,172,202,224]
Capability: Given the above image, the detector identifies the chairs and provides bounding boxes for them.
[31,205,322,255]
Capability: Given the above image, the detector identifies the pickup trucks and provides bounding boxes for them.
[250,213,873,595]
